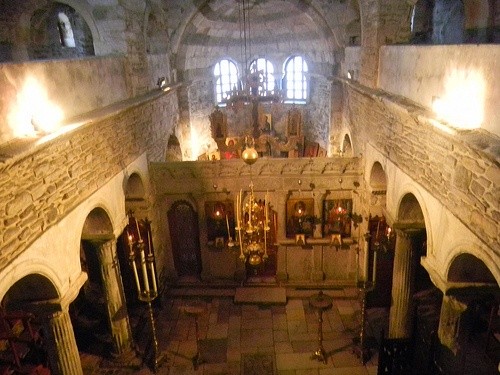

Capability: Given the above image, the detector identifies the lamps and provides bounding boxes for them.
[226,135,269,267]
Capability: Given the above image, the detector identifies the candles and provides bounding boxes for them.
[388,227,391,234]
[216,211,220,216]
[338,207,342,212]
[298,209,301,214]
